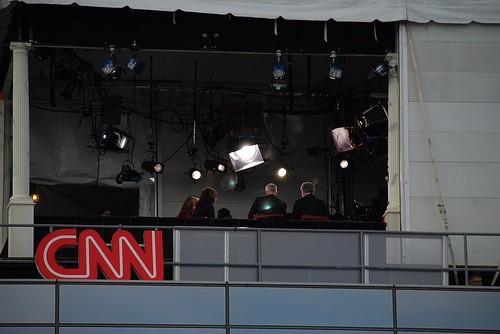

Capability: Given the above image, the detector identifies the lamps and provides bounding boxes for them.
[102,42,123,82]
[368,62,398,83]
[327,50,342,79]
[88,97,289,191]
[270,50,289,89]
[328,105,390,157]
[123,40,145,76]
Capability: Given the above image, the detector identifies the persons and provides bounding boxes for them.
[176,187,218,219]
[290,182,329,220]
[248,182,287,219]
